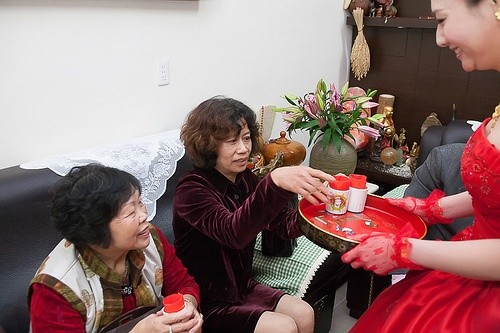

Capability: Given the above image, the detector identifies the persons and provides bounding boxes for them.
[173,95,335,333]
[341,0,500,333]
[27,162,203,333]
[403,143,475,240]
[377,107,405,151]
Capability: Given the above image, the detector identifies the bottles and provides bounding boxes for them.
[368,94,396,163]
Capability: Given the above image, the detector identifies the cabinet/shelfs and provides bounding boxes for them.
[343,0,500,197]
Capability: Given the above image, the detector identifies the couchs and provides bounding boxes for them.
[346,119,482,321]
[0,129,335,333]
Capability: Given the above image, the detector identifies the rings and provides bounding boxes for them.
[170,324,173,333]
[199,313,203,319]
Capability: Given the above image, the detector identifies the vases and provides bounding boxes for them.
[308,138,357,176]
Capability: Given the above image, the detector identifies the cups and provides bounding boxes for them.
[325,185,368,215]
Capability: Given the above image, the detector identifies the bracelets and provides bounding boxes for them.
[400,236,409,260]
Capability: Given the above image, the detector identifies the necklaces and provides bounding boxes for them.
[489,102,500,133]
[120,286,134,294]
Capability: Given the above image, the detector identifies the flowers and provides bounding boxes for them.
[275,79,387,153]
